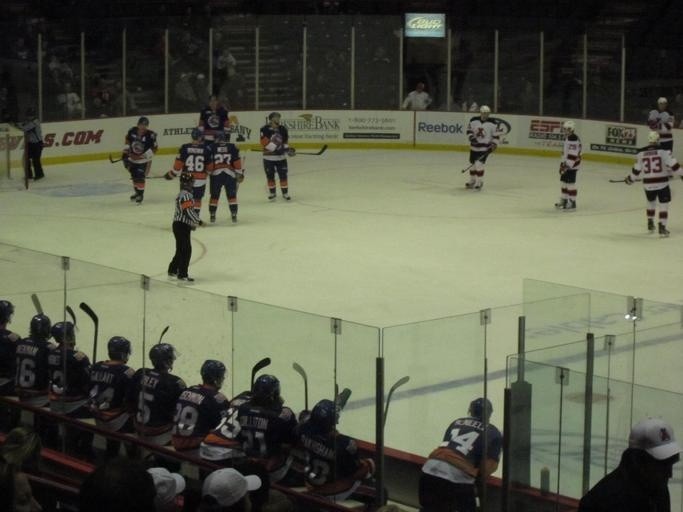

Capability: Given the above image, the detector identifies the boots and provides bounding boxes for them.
[646,219,670,235]
[210,213,216,221]
[554,198,577,209]
[465,177,484,189]
[167,271,196,284]
[265,191,291,200]
[232,213,237,221]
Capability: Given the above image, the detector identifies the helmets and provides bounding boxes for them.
[2,301,14,323]
[189,126,206,138]
[178,171,195,190]
[29,313,76,343]
[479,104,491,115]
[312,397,339,431]
[138,116,151,126]
[469,396,492,419]
[646,130,660,144]
[562,119,576,134]
[148,342,180,367]
[201,359,229,382]
[254,371,280,402]
[107,336,134,361]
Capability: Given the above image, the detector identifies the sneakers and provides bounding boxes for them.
[130,189,144,203]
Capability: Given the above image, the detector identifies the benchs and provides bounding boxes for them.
[1,396,367,511]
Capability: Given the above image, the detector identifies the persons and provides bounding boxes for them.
[465,105,499,192]
[1,300,377,511]
[555,121,583,209]
[400,82,431,112]
[168,173,204,282]
[198,94,231,137]
[121,115,158,202]
[202,132,244,223]
[164,125,213,225]
[625,133,682,236]
[19,110,52,182]
[579,416,682,511]
[646,98,674,151]
[418,398,502,511]
[1,5,248,109]
[257,113,295,199]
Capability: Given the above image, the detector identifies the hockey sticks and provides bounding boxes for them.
[250,144,327,155]
[383,376,409,427]
[65,305,76,327]
[292,362,308,410]
[79,302,99,365]
[462,148,491,173]
[251,357,271,392]
[159,326,169,343]
[32,294,43,316]
[109,154,122,163]
[336,388,351,413]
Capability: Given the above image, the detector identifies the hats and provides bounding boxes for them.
[201,467,264,505]
[627,416,681,464]
[658,96,667,103]
[147,464,187,505]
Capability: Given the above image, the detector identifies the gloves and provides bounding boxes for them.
[487,143,496,153]
[164,171,176,181]
[122,148,130,160]
[274,145,283,154]
[286,148,296,157]
[470,138,480,147]
[235,169,246,184]
[625,175,633,186]
